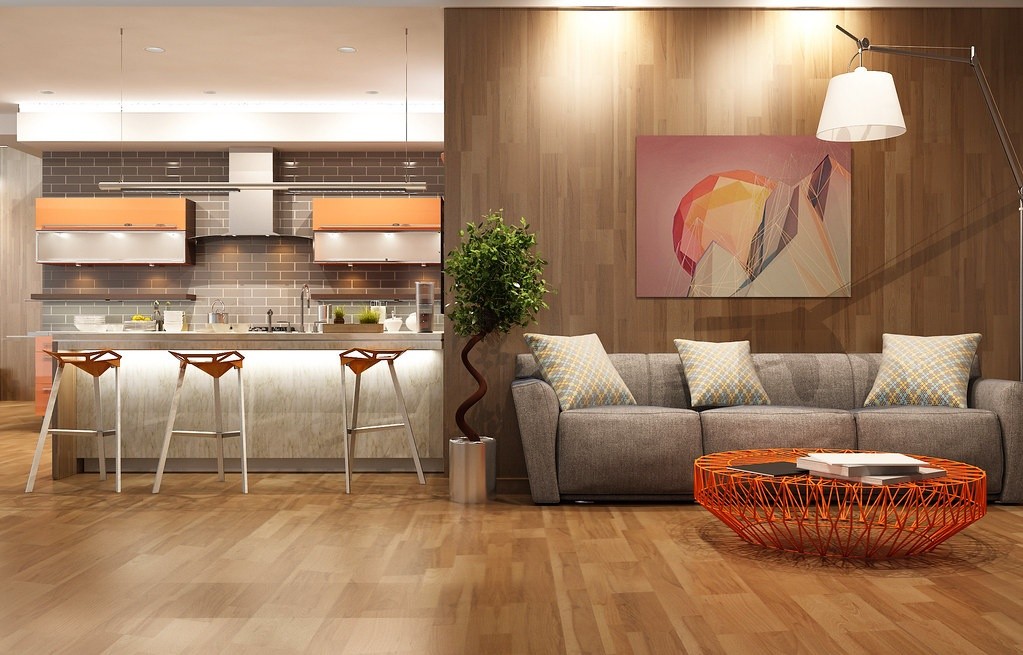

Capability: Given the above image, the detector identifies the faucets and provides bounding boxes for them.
[299,284,311,332]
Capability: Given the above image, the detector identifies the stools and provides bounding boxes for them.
[24,350,122,493]
[338,344,426,494]
[152,351,249,495]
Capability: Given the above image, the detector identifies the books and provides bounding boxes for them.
[794,457,920,476]
[808,451,930,466]
[808,466,948,486]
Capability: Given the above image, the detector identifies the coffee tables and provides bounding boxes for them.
[693,447,987,561]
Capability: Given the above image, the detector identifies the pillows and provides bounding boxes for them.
[863,332,983,409]
[674,338,771,407]
[523,332,639,412]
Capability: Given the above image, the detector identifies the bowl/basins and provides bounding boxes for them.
[164,311,183,332]
[73,316,105,332]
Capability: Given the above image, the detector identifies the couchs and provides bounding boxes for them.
[510,352,1022,506]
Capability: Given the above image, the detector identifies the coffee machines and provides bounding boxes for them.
[415,281,434,333]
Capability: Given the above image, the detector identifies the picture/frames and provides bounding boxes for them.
[635,134,851,299]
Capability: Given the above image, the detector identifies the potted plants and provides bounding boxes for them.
[448,206,559,505]
[334,307,345,324]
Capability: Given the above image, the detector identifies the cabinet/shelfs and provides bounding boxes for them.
[35,336,52,417]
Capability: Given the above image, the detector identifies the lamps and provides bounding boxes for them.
[816,66,907,142]
[98,27,428,195]
